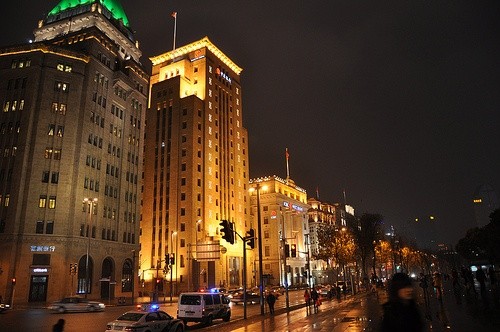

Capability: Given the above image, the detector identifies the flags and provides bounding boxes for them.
[286,151,289,158]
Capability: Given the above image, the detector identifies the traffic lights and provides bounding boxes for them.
[219,220,232,242]
[304,271,307,278]
[291,244,297,258]
[247,229,254,249]
[164,252,175,265]
[155,278,160,286]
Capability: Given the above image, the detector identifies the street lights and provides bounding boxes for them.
[170,231,178,303]
[83,198,97,300]
[250,183,268,315]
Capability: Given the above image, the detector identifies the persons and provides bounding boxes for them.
[267,292,275,315]
[330,284,347,299]
[304,290,311,308]
[461,265,497,295]
[311,288,319,308]
[53,319,65,332]
[419,272,442,305]
[382,272,426,332]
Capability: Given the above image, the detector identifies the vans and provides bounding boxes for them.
[177,292,230,327]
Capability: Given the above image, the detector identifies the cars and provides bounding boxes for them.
[48,297,105,313]
[311,281,363,296]
[227,289,281,304]
[105,304,184,332]
